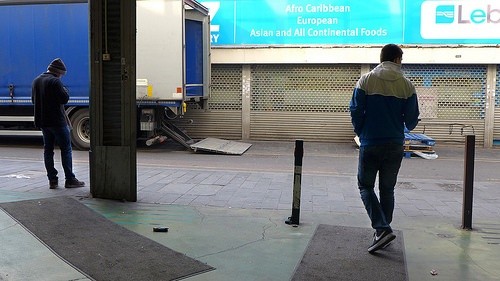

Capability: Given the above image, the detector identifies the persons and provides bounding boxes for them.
[32,58,85,189]
[350,43,419,253]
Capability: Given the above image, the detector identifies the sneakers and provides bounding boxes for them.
[368,226,396,252]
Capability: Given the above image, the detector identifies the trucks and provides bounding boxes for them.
[0,0,211,152]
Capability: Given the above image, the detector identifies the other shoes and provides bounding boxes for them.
[65,175,85,187]
[50,178,58,188]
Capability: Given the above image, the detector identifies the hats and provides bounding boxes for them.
[47,57,67,74]
